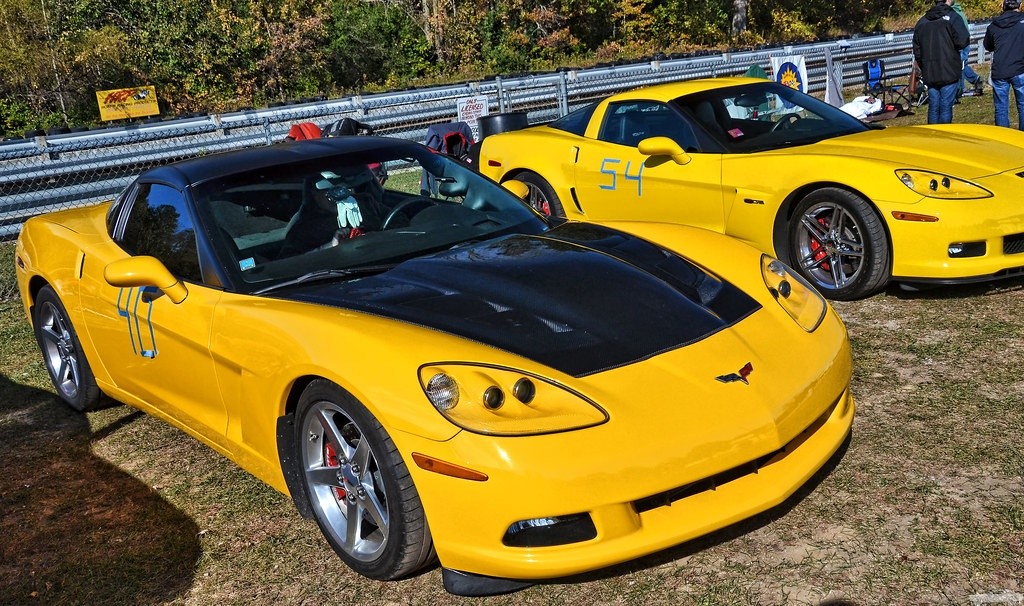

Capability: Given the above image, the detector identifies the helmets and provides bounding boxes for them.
[1003,0,1020,11]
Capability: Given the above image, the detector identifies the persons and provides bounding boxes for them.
[914,1,1024,132]
[284,182,408,251]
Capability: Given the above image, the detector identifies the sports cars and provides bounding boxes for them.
[477,76,1024,303]
[12,125,858,600]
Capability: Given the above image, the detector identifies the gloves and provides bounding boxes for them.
[337,195,363,229]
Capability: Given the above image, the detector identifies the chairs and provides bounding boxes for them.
[862,58,912,112]
[613,101,731,154]
[476,110,529,143]
[283,165,410,258]
[421,122,477,202]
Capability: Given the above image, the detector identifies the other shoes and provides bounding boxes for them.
[975,76,985,95]
